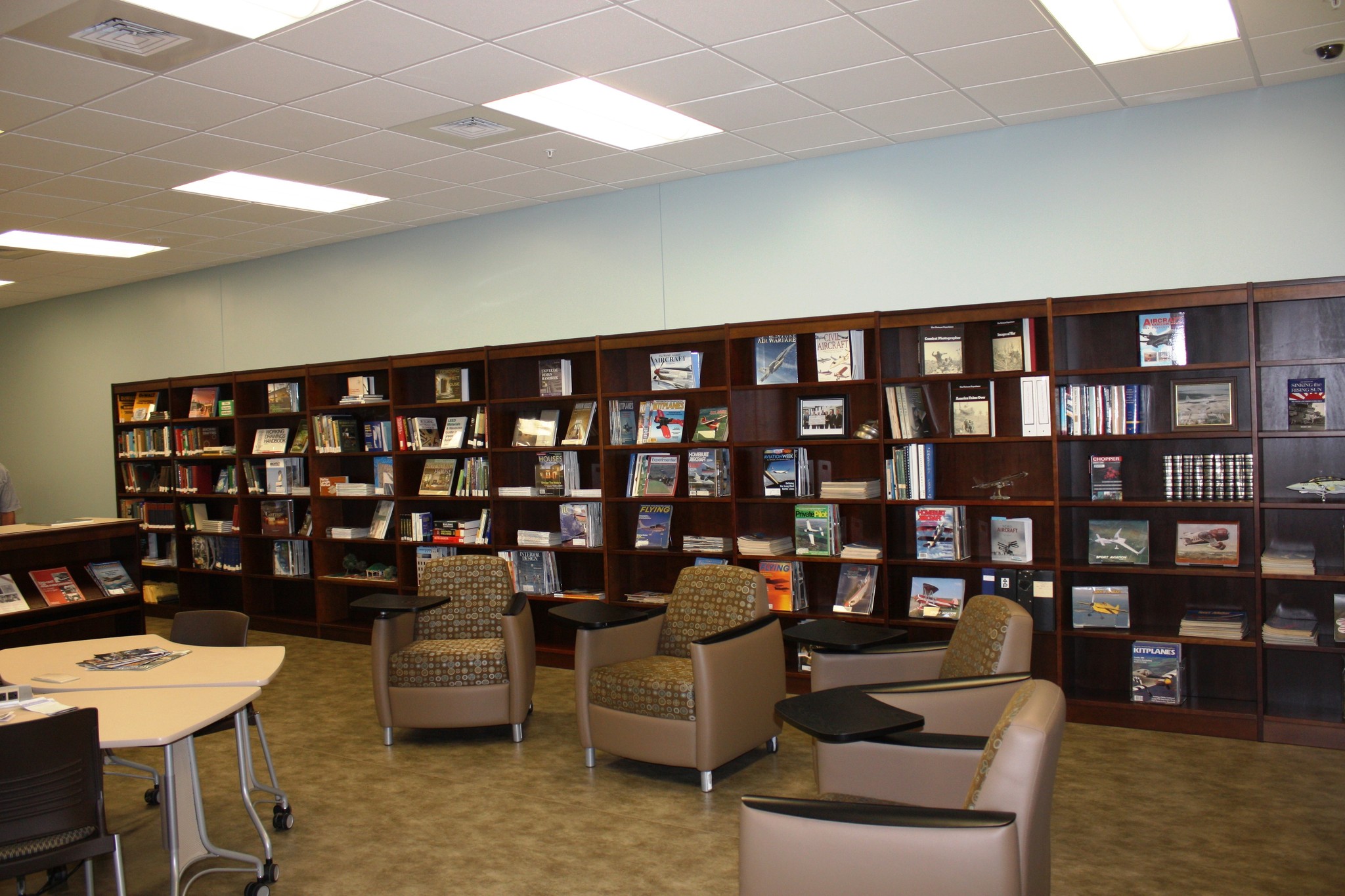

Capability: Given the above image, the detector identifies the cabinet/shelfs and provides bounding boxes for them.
[597,325,738,613]
[304,356,398,645]
[483,334,603,671]
[724,311,884,693]
[879,300,1060,688]
[1048,282,1265,747]
[0,516,145,650]
[232,364,320,639]
[168,371,243,620]
[1248,275,1345,752]
[389,347,490,598]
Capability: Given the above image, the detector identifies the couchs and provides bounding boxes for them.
[372,554,537,746]
[573,563,788,793]
[739,678,1066,896]
[811,593,1034,736]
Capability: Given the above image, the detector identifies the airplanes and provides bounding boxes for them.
[654,408,684,438]
[917,523,953,548]
[637,519,669,537]
[699,414,728,432]
[803,520,825,546]
[816,334,852,380]
[1093,528,1147,556]
[1140,329,1177,348]
[1180,528,1230,551]
[648,469,675,488]
[759,343,795,383]
[914,583,960,612]
[1133,666,1178,697]
[1075,595,1129,620]
[1182,395,1218,404]
[843,568,873,607]
[1100,466,1119,479]
[651,356,693,381]
[996,539,1019,555]
[770,466,791,475]
[1285,475,1345,501]
[764,576,789,587]
[773,585,790,592]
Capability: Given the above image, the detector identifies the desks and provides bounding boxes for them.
[546,600,647,630]
[110,378,180,622]
[774,685,925,744]
[781,618,908,652]
[347,592,453,613]
[0,686,269,896]
[0,634,286,885]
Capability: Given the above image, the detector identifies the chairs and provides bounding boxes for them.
[169,610,295,830]
[0,706,117,896]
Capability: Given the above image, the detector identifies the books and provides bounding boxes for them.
[395,366,492,587]
[311,376,395,539]
[737,330,880,617]
[607,349,731,603]
[1054,308,1253,710]
[174,386,242,573]
[1259,374,1345,646]
[242,382,312,577]
[117,392,179,604]
[75,646,192,672]
[26,518,94,526]
[496,358,605,601]
[883,318,1057,635]
[1,558,139,615]
